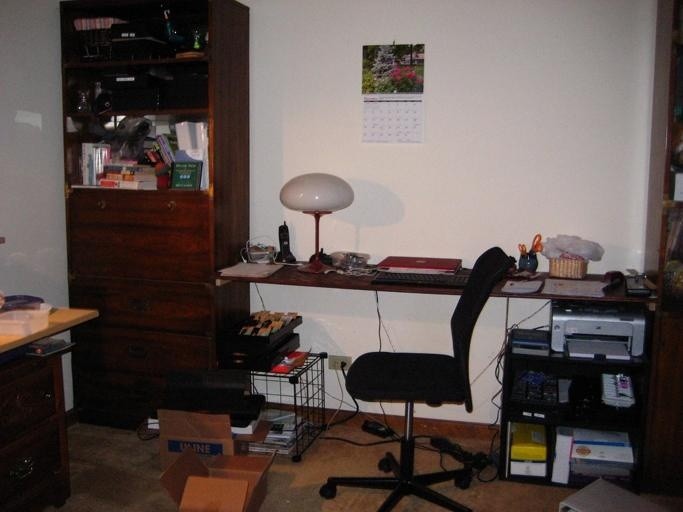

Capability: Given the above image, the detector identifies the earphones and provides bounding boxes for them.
[241,256,247,263]
[298,263,303,266]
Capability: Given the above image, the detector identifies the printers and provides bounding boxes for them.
[551,300,646,361]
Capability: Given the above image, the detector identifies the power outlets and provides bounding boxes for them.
[328,355,353,371]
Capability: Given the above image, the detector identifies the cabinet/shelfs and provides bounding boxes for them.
[502,332,642,487]
[58,0,249,189]
[62,189,249,433]
[641,0,681,495]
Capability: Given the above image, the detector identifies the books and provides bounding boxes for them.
[0,310,49,337]
[78,140,111,186]
[247,416,308,455]
[146,132,178,174]
[602,371,636,408]
[167,162,202,191]
[95,163,158,192]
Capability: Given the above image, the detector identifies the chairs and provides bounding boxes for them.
[320,243,518,512]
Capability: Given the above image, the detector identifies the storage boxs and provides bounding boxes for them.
[510,422,546,480]
[159,445,278,512]
[155,408,273,470]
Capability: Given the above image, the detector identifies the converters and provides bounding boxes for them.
[362,420,391,438]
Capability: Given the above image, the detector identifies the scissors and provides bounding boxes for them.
[519,244,527,255]
[530,234,543,253]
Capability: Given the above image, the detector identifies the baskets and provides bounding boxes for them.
[548,256,588,280]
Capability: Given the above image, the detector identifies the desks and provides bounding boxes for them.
[221,252,656,495]
[0,292,102,512]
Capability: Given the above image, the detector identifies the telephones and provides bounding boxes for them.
[276,221,296,263]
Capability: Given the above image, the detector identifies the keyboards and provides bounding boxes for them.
[371,271,470,287]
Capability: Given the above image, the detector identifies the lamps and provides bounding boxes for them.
[277,172,354,274]
